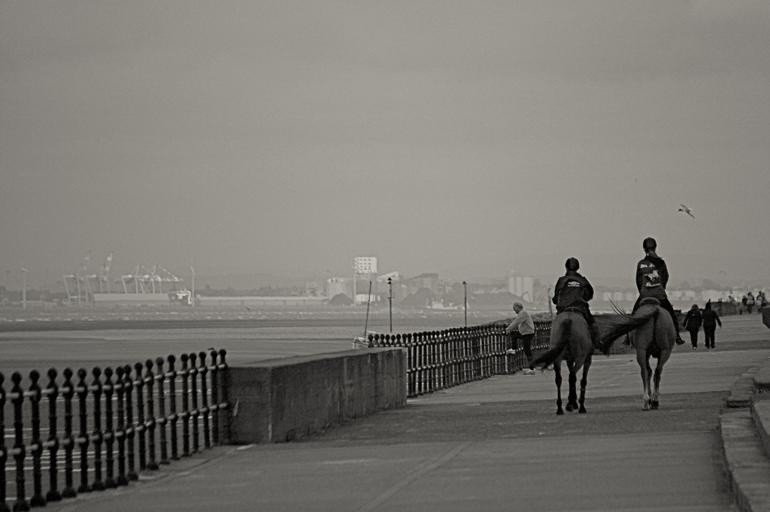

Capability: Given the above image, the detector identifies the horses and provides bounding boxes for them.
[600,297,677,411]
[529,312,594,414]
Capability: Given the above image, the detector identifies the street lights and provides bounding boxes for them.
[387,277,393,331]
[463,281,466,328]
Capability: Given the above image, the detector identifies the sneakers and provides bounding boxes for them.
[507,348,536,375]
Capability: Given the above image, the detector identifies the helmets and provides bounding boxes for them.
[643,237,657,248]
[565,257,579,270]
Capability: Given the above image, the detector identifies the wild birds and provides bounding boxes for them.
[678,204,697,218]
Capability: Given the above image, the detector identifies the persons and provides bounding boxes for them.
[555,255,597,347]
[740,289,770,330]
[682,302,719,349]
[505,302,538,375]
[628,237,687,350]
